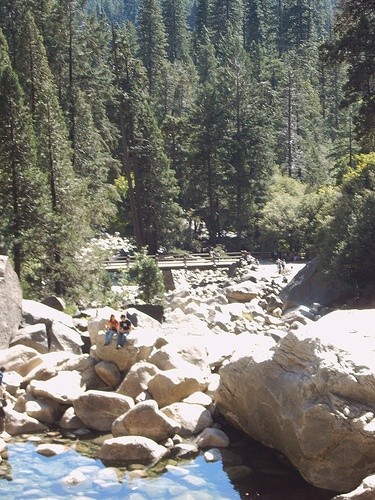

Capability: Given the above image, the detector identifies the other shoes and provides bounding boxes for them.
[104,342,109,345]
[116,345,122,349]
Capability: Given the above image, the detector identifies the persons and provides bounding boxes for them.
[282,275,287,283]
[0,366,7,394]
[276,258,285,274]
[0,405,7,431]
[116,314,132,350]
[104,314,119,347]
[271,250,299,261]
